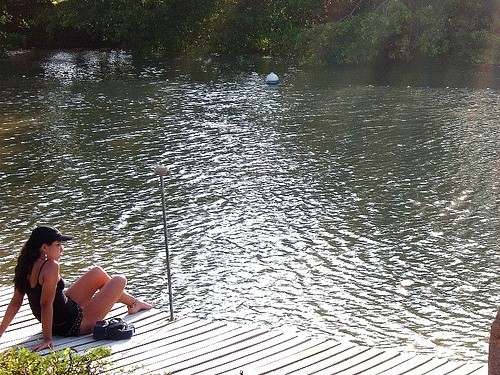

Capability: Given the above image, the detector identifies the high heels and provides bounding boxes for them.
[94,317,123,340]
[105,321,136,340]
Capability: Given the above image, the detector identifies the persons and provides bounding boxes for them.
[0,226,156,353]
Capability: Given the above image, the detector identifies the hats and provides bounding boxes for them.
[31,225,73,244]
